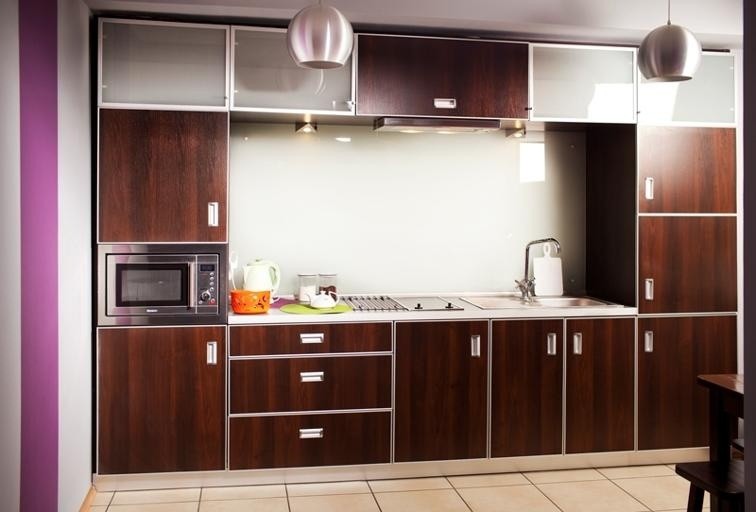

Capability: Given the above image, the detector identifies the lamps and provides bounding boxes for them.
[505,127,527,140]
[373,118,500,133]
[294,122,318,133]
[285,0,353,67]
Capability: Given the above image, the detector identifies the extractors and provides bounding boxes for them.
[372,119,503,137]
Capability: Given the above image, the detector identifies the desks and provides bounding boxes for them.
[698,374,744,460]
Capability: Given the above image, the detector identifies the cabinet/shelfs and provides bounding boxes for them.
[637,121,744,465]
[94,326,224,487]
[394,319,489,478]
[491,319,636,471]
[96,107,231,244]
[225,323,390,482]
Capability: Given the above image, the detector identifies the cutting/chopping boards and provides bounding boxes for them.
[532,243,566,297]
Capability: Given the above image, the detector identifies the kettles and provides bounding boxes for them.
[240,260,282,300]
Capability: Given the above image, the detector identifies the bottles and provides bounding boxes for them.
[318,272,338,300]
[299,273,316,304]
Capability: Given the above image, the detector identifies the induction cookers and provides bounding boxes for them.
[343,295,464,313]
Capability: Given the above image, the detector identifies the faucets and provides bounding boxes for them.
[515,238,561,300]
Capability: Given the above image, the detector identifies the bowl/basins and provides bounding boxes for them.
[231,291,270,313]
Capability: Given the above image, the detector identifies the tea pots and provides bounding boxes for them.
[302,290,341,309]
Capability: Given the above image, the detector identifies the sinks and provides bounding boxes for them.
[535,296,625,308]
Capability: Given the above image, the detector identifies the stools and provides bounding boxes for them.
[675,459,745,512]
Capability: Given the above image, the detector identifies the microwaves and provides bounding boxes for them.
[95,243,226,326]
